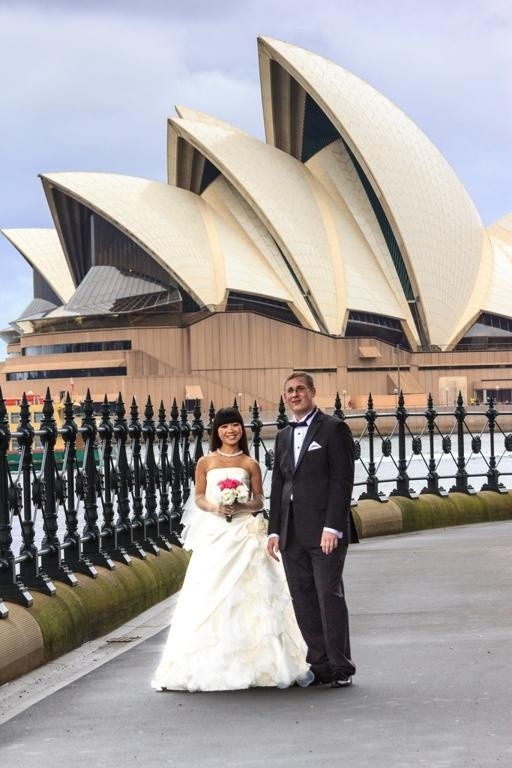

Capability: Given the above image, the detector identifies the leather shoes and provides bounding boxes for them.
[295,672,351,688]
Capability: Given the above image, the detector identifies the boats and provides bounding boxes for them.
[8,402,103,470]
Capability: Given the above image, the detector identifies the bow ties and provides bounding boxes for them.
[288,421,308,427]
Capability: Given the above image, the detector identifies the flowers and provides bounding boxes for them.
[217,478,249,522]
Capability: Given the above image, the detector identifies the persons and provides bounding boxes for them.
[471,397,480,405]
[267,372,360,686]
[151,407,316,692]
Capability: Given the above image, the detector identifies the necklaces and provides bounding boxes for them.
[217,448,244,457]
